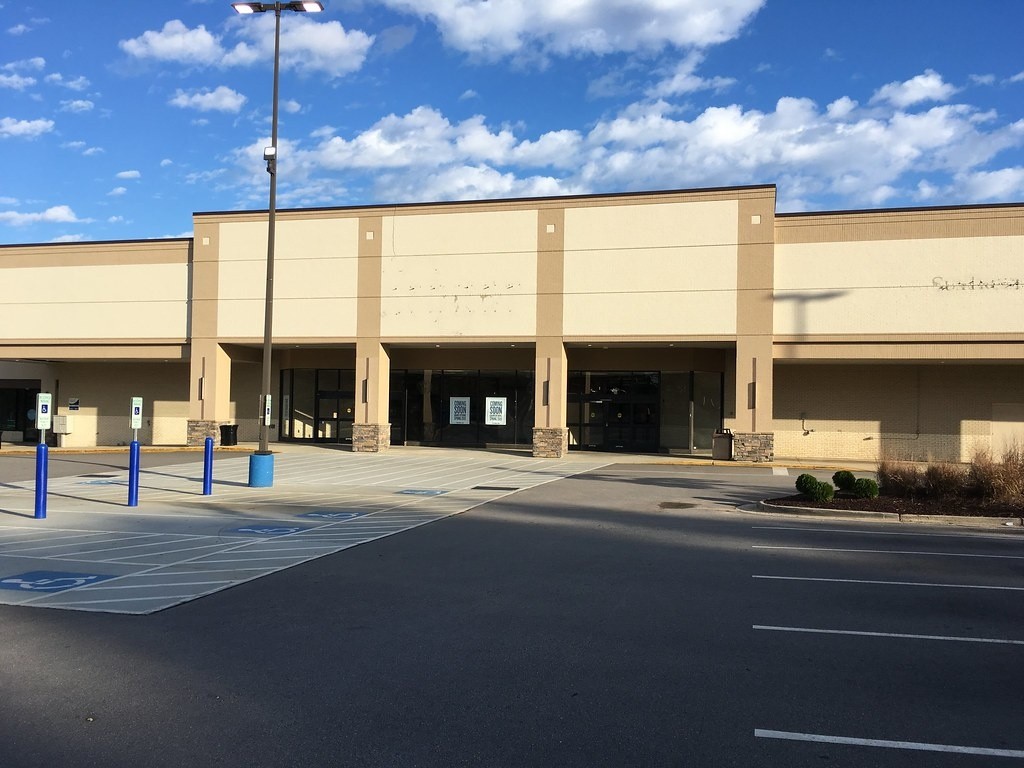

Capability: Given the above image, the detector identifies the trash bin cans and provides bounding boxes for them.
[712,429,733,460]
[220,424,239,446]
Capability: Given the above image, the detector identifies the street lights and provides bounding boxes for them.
[229,0,324,484]
